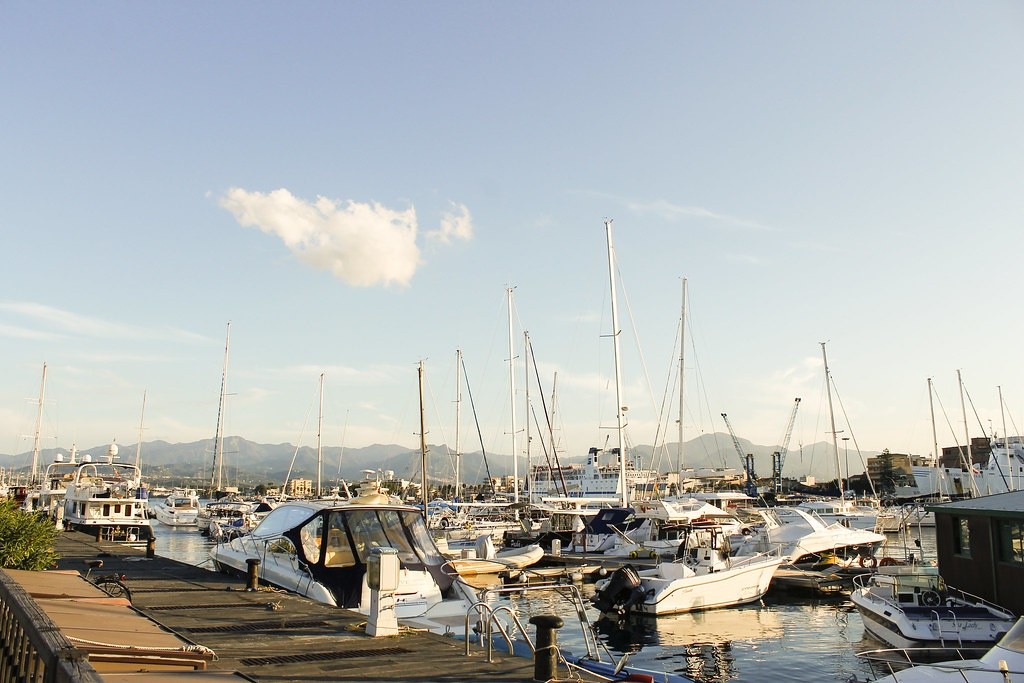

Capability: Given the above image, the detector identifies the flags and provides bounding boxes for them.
[973,468,979,475]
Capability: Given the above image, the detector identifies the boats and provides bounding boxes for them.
[211,490,510,641]
[598,545,781,614]
[870,616,1024,683]
[853,572,1020,662]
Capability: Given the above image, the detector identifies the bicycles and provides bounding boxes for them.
[47,561,131,604]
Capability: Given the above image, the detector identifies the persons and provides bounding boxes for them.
[135,481,147,498]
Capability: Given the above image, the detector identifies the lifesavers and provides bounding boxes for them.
[858,554,878,568]
[440,520,449,529]
[879,557,897,566]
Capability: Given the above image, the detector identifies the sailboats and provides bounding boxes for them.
[5,218,1023,549]
